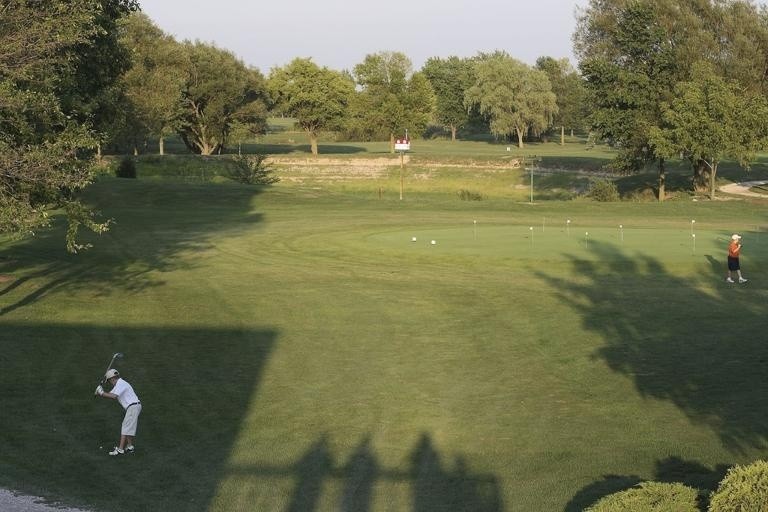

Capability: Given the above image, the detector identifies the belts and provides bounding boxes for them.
[129,402,140,406]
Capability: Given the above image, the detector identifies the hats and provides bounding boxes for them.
[104,369,120,383]
[732,234,741,239]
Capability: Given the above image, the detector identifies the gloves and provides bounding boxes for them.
[95,385,104,397]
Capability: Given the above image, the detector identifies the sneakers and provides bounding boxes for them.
[109,447,125,456]
[739,277,747,283]
[124,445,135,453]
[726,277,734,283]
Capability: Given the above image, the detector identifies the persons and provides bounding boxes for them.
[96,368,143,456]
[728,233,748,284]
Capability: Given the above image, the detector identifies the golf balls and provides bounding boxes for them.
[100,446,104,449]
[411,236,417,242]
[431,240,436,244]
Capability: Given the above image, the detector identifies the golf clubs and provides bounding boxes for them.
[94,352,125,398]
[716,237,744,249]
